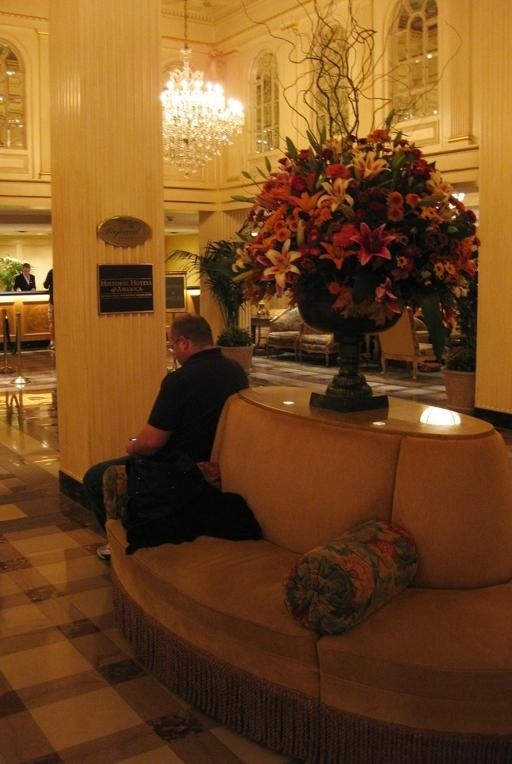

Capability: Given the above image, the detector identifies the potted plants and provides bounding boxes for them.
[166,237,258,374]
[440,346,477,411]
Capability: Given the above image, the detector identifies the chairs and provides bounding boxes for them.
[378,305,435,380]
[269,300,341,366]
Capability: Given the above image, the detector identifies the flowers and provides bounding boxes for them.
[229,131,481,364]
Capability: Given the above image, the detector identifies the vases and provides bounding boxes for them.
[297,278,416,412]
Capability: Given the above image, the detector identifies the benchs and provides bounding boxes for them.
[105,384,510,760]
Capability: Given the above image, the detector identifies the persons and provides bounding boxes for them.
[83,312,249,561]
[43,268,56,350]
[15,263,36,293]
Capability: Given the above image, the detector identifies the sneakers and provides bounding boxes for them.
[97,543,111,560]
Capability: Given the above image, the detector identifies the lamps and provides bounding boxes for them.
[161,2,244,179]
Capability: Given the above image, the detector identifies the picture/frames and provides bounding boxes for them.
[95,262,157,317]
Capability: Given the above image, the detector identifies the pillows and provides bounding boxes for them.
[104,460,220,521]
[285,517,419,634]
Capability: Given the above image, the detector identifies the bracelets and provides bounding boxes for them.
[132,440,139,452]
